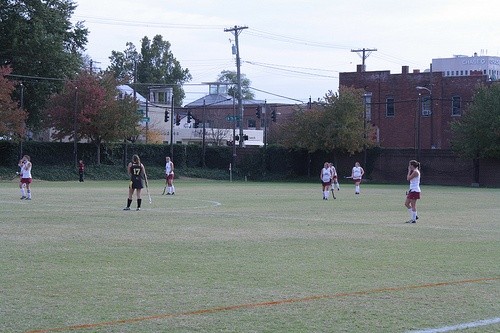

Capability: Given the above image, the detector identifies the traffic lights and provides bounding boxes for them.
[176,114,181,126]
[235,136,240,141]
[187,110,193,123]
[255,105,260,119]
[244,136,248,140]
[164,111,170,122]
[271,110,277,123]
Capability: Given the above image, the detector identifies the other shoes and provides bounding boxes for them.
[406,219,416,224]
[167,192,171,195]
[21,196,26,199]
[123,207,130,210]
[172,192,175,195]
[25,197,31,200]
[416,216,419,220]
[136,208,140,210]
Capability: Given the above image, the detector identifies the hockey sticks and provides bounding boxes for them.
[401,173,410,188]
[342,175,362,179]
[144,173,152,204]
[160,180,167,196]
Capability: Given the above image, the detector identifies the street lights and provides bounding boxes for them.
[415,86,433,149]
[221,70,244,149]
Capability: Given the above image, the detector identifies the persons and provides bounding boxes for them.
[405,160,421,224]
[320,161,339,200]
[165,156,175,195]
[351,162,365,194]
[78,160,84,182]
[122,155,144,210]
[16,155,32,200]
